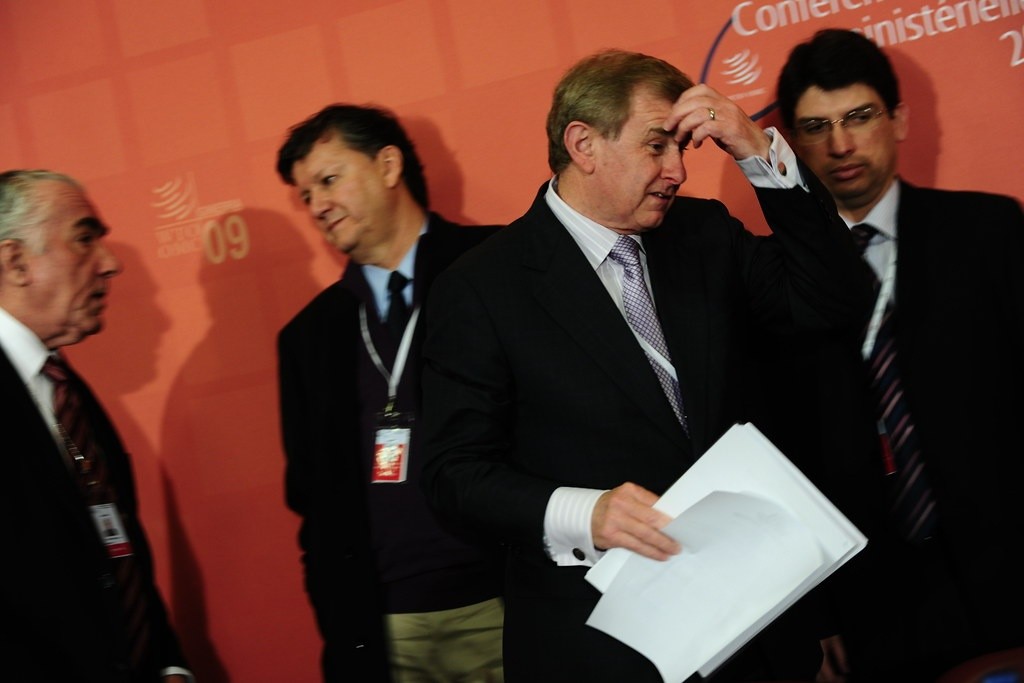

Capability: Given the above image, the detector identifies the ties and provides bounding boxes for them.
[850,224,938,552]
[609,236,691,432]
[40,358,99,487]
[383,271,410,373]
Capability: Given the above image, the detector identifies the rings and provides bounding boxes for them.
[706,108,716,119]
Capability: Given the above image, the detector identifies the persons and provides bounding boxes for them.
[0,169,206,683]
[776,29,1024,682]
[272,101,521,683]
[512,49,881,682]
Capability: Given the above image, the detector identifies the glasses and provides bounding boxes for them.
[791,104,892,145]
